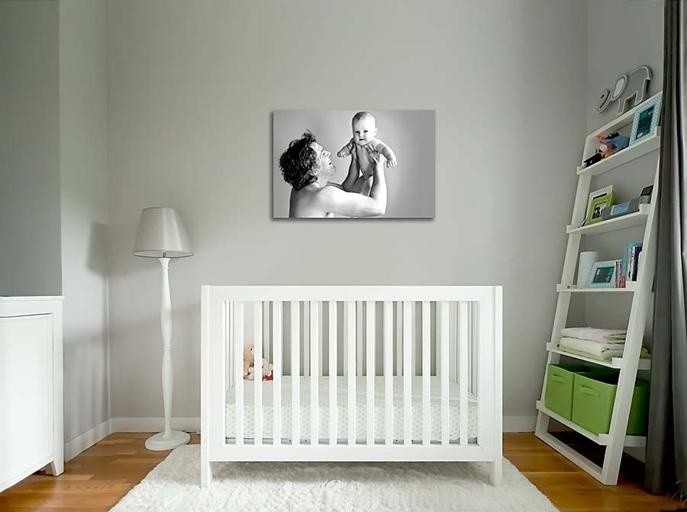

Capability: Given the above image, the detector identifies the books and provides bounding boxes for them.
[611,239,643,289]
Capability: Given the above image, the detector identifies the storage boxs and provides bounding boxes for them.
[572,371,651,437]
[545,362,608,421]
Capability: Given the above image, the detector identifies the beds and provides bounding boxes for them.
[201,285,503,493]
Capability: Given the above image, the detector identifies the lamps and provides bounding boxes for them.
[132,207,194,451]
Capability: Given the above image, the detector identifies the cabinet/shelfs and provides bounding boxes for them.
[533,88,662,487]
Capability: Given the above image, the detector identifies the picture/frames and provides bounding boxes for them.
[588,260,618,288]
[586,184,612,218]
[588,193,613,224]
[628,93,661,146]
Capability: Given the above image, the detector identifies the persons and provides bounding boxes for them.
[333,109,397,198]
[276,127,388,219]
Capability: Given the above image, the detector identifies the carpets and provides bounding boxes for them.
[105,444,560,512]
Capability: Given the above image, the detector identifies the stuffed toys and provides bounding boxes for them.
[242,342,274,381]
